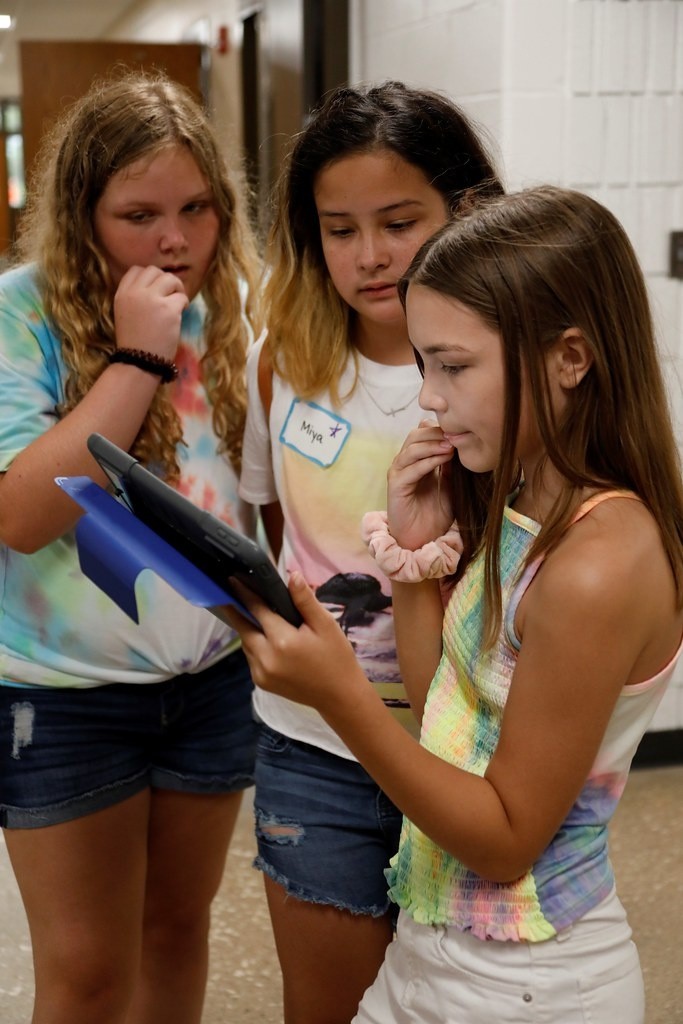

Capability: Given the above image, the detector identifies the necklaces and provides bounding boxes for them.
[347,343,422,417]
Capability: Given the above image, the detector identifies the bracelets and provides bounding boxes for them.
[106,345,180,385]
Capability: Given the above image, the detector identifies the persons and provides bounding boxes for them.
[0,74,271,1023]
[215,184,682,1024]
[238,129,513,1022]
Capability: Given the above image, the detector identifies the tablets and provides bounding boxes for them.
[85,430,305,631]
